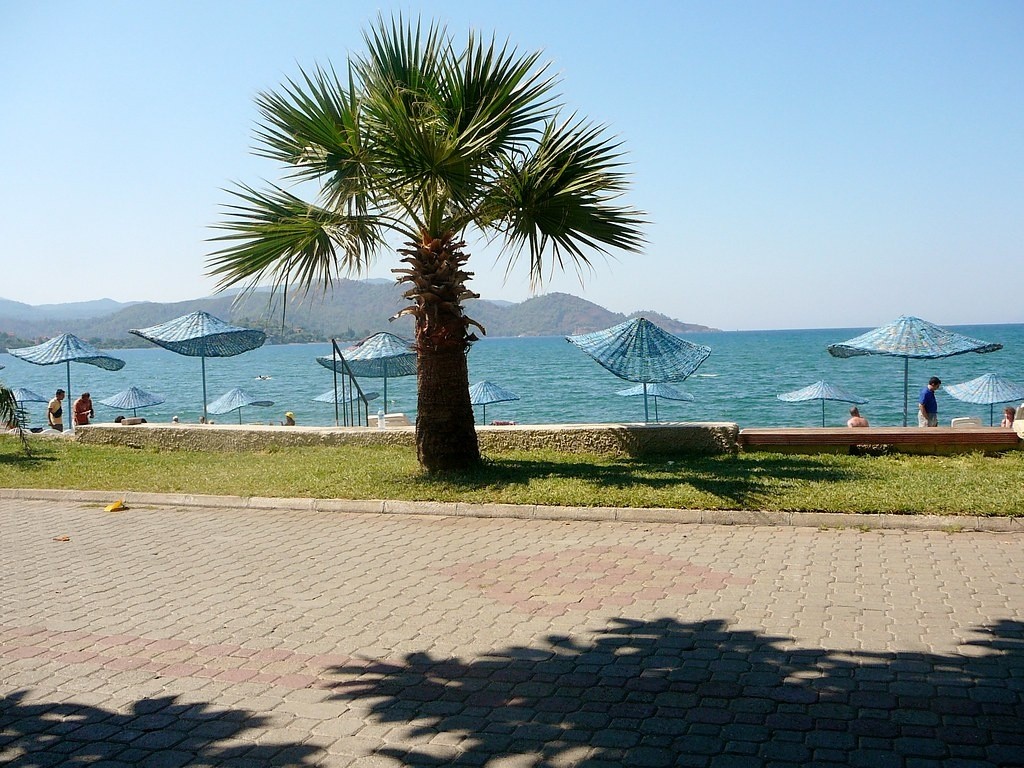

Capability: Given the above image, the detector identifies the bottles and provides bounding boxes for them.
[378,408,385,429]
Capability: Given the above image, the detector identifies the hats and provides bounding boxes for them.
[1004,406,1016,415]
[285,412,295,421]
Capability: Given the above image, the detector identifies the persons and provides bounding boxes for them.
[73,392,94,426]
[199,416,205,424]
[847,407,868,427]
[1001,407,1015,428]
[172,416,178,423]
[281,412,296,426]
[47,389,66,432]
[115,416,125,423]
[918,377,941,427]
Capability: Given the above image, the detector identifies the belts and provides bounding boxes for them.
[926,411,938,414]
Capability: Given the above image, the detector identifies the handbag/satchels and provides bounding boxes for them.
[53,407,62,418]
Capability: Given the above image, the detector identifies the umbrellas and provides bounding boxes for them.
[828,315,1003,427]
[566,318,711,421]
[316,332,419,413]
[0,333,165,428]
[468,381,519,426]
[204,387,274,424]
[776,380,868,427]
[943,373,1024,427]
[130,311,266,425]
[313,384,380,426]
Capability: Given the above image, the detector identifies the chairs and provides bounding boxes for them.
[367,412,414,428]
[950,416,983,429]
